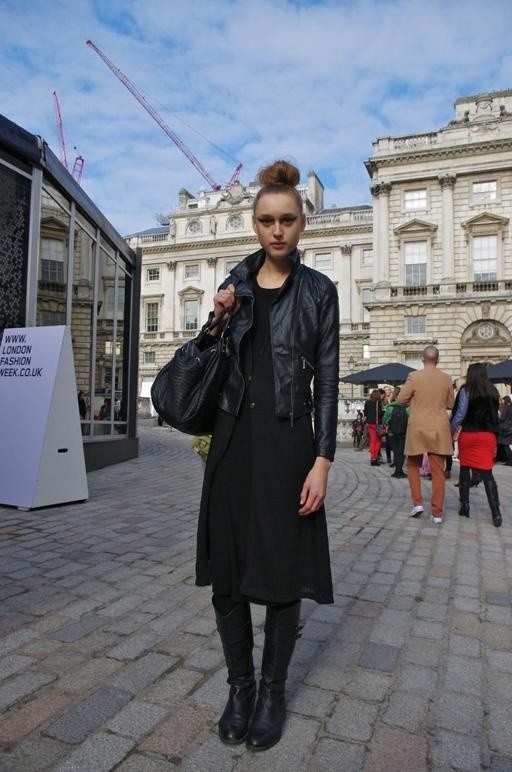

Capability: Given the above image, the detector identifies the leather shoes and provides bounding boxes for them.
[444,470,451,478]
[370,460,407,479]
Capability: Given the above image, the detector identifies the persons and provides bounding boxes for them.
[191,161,339,752]
[451,363,502,527]
[397,346,455,524]
[352,386,512,478]
[78,382,117,420]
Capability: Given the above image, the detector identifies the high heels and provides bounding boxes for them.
[469,476,480,487]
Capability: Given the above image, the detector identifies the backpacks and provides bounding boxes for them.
[388,405,407,436]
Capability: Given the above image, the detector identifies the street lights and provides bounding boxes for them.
[347,354,357,398]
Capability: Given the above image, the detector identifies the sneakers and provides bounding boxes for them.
[430,515,442,524]
[409,505,424,517]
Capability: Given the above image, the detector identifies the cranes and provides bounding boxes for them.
[86,40,243,192]
[50,89,84,184]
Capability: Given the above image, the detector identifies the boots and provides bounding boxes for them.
[485,479,502,527]
[246,600,301,752]
[211,596,257,746]
[459,479,470,518]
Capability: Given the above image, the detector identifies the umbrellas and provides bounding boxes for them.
[339,362,418,387]
[485,360,512,384]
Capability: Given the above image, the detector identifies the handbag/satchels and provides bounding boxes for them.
[376,424,386,437]
[147,284,241,437]
[452,425,462,441]
